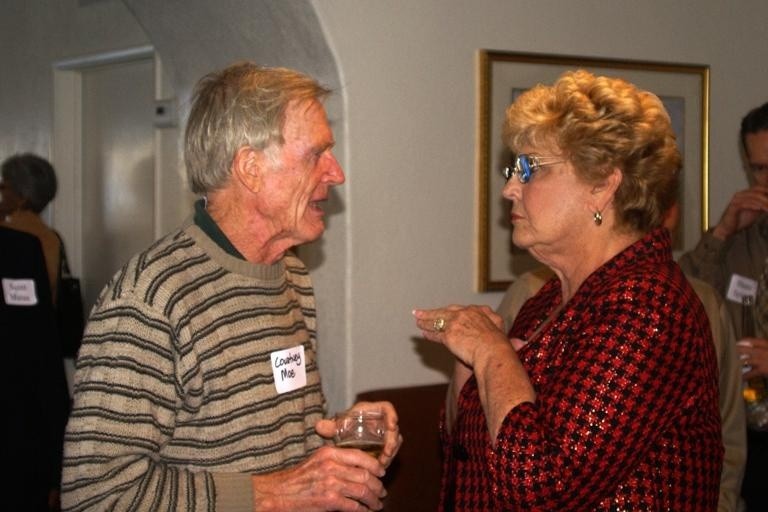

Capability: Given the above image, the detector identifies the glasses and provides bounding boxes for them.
[501,153,572,185]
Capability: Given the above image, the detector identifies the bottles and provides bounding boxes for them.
[740,295,766,407]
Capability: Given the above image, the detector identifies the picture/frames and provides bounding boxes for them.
[473,46,711,294]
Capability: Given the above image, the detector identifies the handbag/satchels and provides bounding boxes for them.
[53,228,85,359]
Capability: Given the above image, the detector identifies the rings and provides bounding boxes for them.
[434,316,446,333]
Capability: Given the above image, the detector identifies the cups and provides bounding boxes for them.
[333,410,386,459]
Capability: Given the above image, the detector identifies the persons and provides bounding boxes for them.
[50,59,408,510]
[0,148,85,510]
[409,68,724,510]
[433,170,749,509]
[675,103,768,438]
